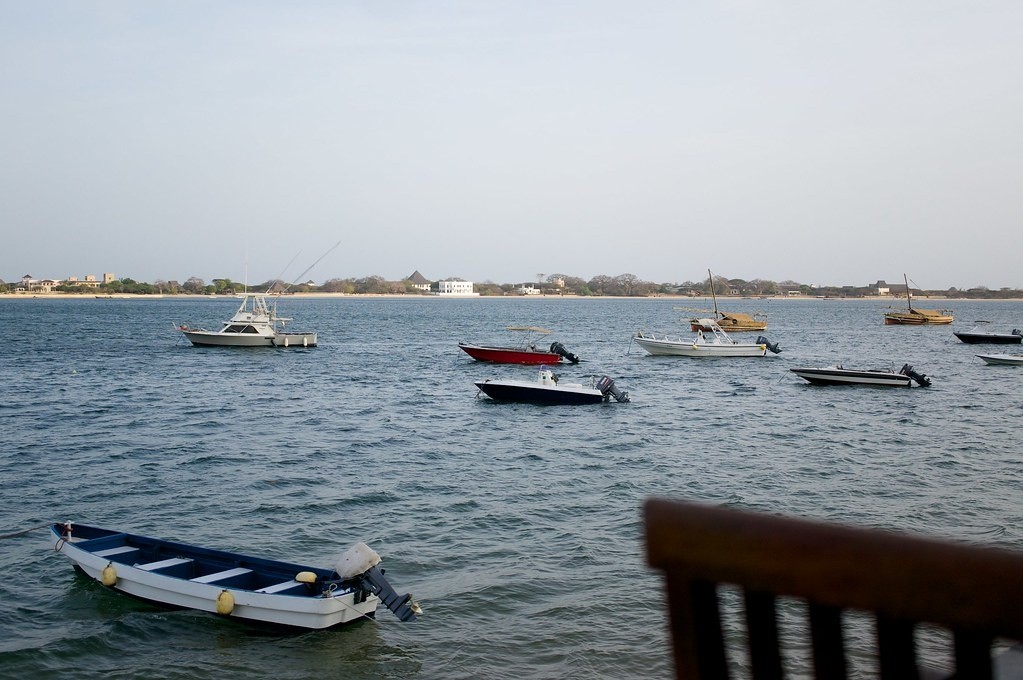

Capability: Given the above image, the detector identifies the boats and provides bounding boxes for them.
[472,363,631,405]
[457,326,581,364]
[691,269,768,332]
[952,325,1023,344]
[974,352,1023,366]
[171,261,317,348]
[633,318,782,357]
[50,518,425,631]
[789,362,932,388]
[884,272,954,326]
